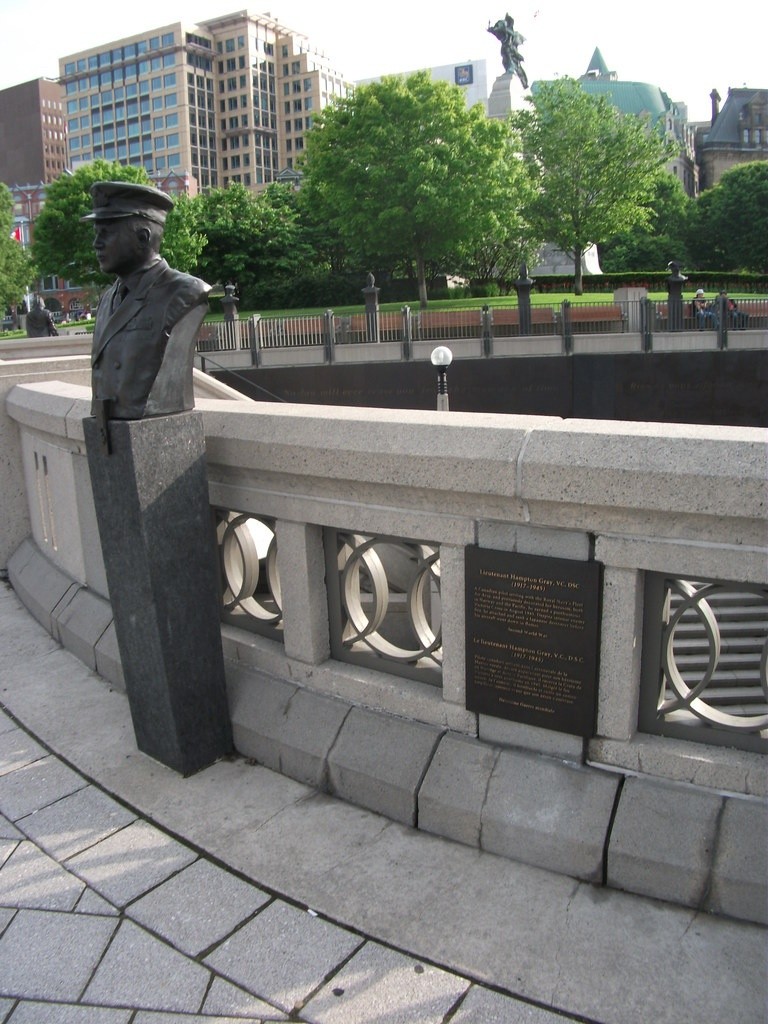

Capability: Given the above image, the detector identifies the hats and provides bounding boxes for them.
[696,288,704,295]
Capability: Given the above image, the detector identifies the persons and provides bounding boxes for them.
[692,289,749,331]
[361,272,380,312]
[514,262,536,303]
[220,284,239,319]
[665,261,688,298]
[25,295,60,337]
[79,181,215,421]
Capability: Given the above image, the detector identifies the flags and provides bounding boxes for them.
[10,227,22,242]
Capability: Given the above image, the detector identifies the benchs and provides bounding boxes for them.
[492,308,558,340]
[570,304,629,335]
[197,326,228,350]
[284,318,342,344]
[417,309,484,340]
[347,315,408,342]
[656,303,711,332]
[232,322,274,347]
[735,301,768,329]
